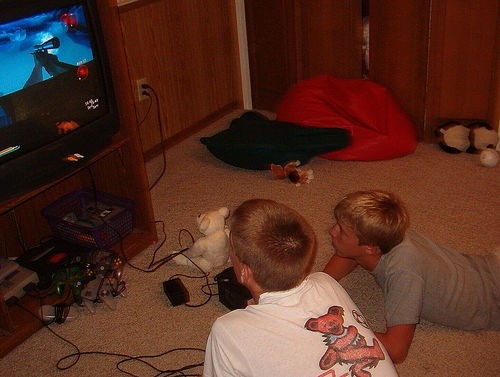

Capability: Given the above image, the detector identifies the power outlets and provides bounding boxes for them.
[136,78,151,104]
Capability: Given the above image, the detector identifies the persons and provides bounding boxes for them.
[323,189,500,365]
[201,198,398,377]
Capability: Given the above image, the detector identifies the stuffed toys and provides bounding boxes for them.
[269,159,315,187]
[439,123,500,155]
[172,205,236,270]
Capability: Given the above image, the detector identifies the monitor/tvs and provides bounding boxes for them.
[0,0,120,203]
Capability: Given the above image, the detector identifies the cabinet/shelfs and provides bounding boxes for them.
[0,0,163,358]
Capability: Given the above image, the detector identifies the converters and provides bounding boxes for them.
[164,278,190,307]
